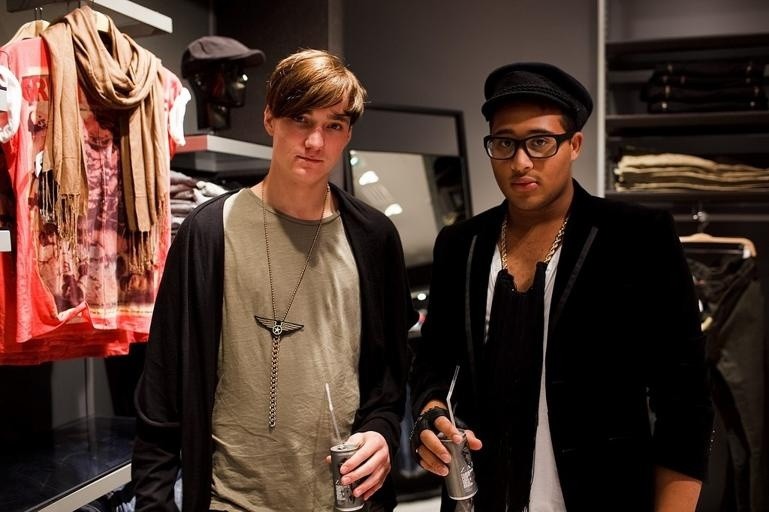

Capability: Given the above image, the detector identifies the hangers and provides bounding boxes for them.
[0,0,178,84]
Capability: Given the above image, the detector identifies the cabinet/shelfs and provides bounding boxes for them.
[605,32,767,204]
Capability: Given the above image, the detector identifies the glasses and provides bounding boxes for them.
[484,132,570,160]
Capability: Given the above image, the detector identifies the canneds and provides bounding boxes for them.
[329,443,363,511]
[435,430,481,501]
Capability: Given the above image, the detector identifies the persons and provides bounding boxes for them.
[182,37,265,132]
[411,61,715,511]
[131,50,420,510]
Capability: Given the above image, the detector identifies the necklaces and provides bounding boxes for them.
[256,186,329,427]
[501,206,568,275]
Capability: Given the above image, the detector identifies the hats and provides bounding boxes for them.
[182,36,264,78]
[482,62,593,125]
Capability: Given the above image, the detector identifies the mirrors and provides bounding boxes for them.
[340,103,475,501]
